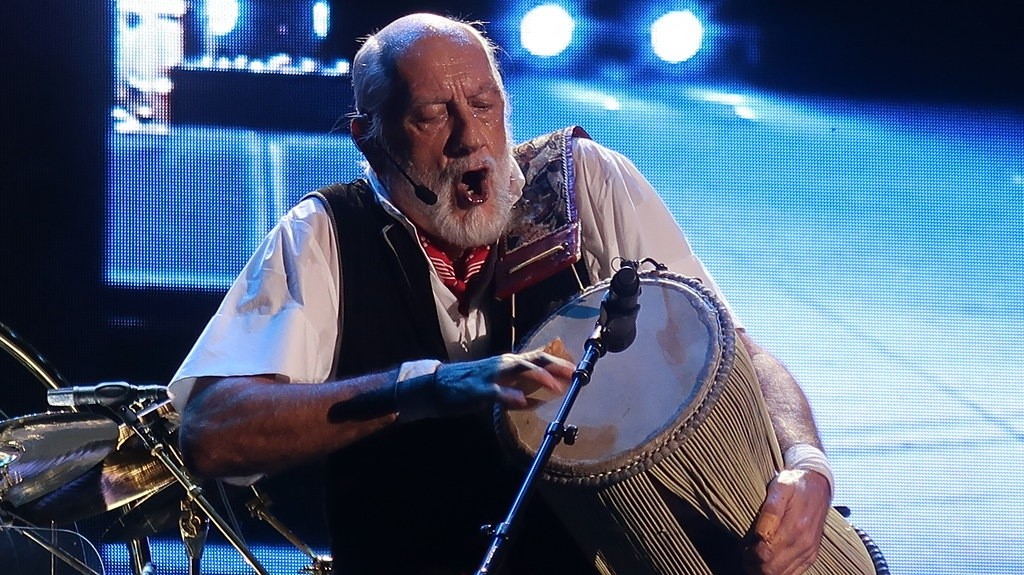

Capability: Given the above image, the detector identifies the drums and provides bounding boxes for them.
[489,267,890,575]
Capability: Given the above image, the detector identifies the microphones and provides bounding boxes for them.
[375,140,439,207]
[47,382,167,406]
[595,267,641,352]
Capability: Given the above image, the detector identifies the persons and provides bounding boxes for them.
[167,13,835,575]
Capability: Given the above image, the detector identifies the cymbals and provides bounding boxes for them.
[0,407,120,509]
[17,396,188,529]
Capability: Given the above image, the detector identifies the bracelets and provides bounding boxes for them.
[393,359,443,421]
[784,444,835,503]
